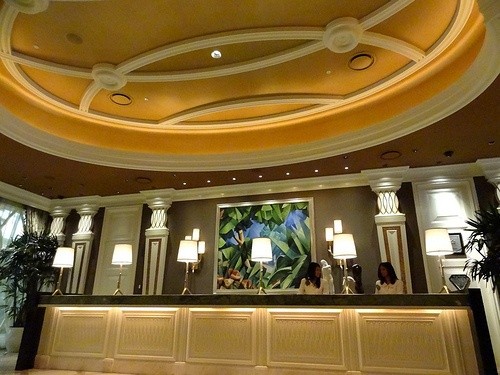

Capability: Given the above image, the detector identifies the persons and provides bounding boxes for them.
[375,262,408,295]
[298,261,330,295]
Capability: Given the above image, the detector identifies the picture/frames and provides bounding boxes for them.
[213,196,316,292]
[449,231,465,257]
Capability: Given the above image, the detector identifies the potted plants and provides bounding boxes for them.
[0,229,64,354]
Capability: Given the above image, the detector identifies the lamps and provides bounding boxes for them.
[249,236,273,294]
[330,230,358,295]
[176,239,200,295]
[49,246,75,295]
[325,220,343,261]
[183,228,206,272]
[110,244,135,294]
[423,228,455,294]
